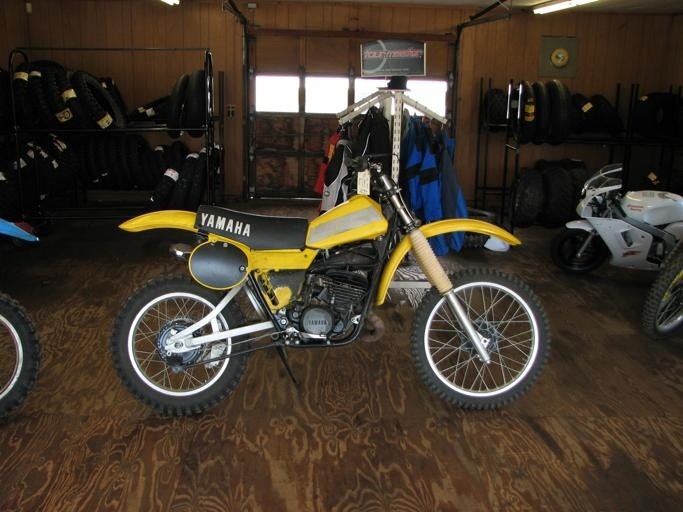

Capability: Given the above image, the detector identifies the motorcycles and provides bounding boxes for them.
[107,109,551,419]
[554,161,682,277]
[642,240,682,342]
[0,212,49,422]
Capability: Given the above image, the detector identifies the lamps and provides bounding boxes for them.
[530,1,599,16]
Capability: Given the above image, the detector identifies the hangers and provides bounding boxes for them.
[335,95,446,133]
